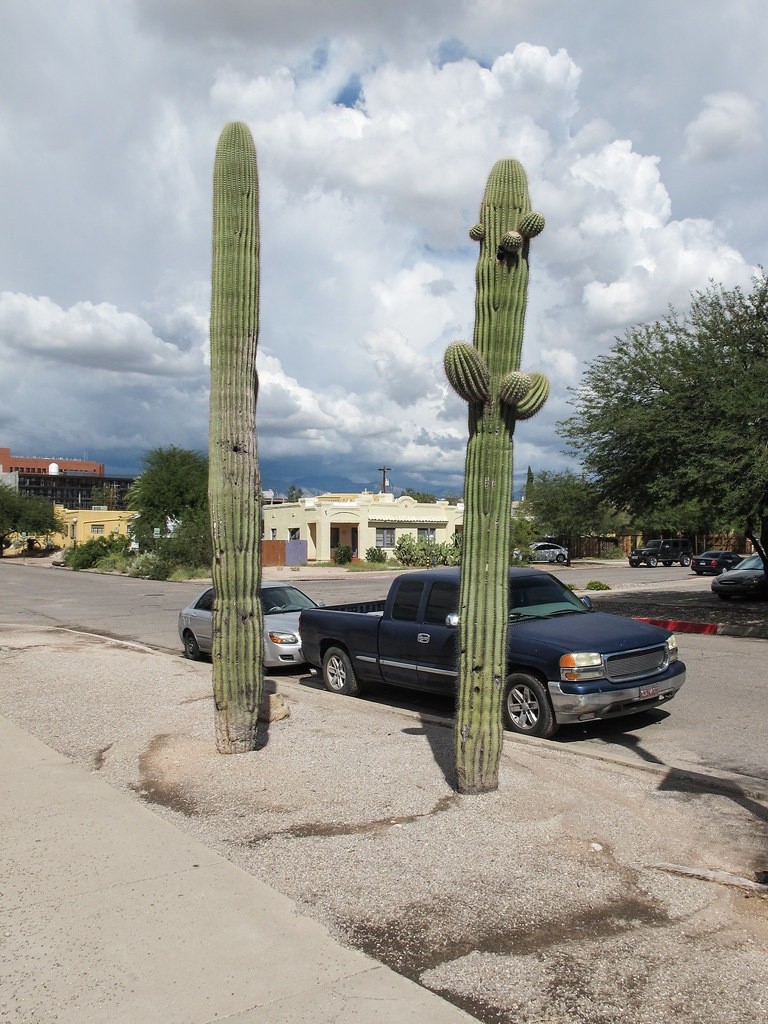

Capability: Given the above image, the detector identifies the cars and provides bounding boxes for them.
[512,542,568,564]
[177,581,321,675]
[711,553,768,599]
[691,550,743,575]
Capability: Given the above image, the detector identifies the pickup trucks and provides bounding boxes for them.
[297,567,687,747]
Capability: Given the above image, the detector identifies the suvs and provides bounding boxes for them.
[628,539,694,568]
